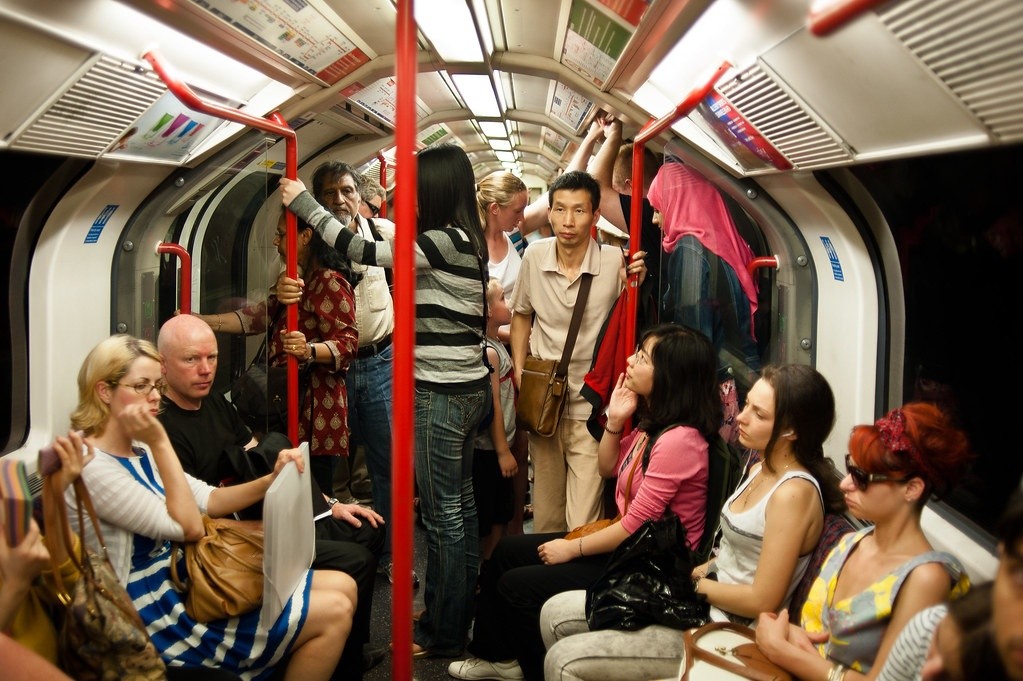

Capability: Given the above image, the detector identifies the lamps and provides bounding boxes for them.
[390,0,521,176]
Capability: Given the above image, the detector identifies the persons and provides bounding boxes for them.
[62,331,358,681]
[277,112,657,587]
[540,365,847,681]
[282,142,492,656]
[166,196,356,510]
[611,401,1023,681]
[0,432,118,681]
[649,160,757,354]
[146,313,385,680]
[438,323,726,681]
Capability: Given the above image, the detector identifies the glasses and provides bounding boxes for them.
[275,231,286,238]
[635,343,654,371]
[948,579,1011,681]
[110,383,167,396]
[845,453,908,493]
[363,199,380,215]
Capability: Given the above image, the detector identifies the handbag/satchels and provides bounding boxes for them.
[585,518,710,631]
[231,430,338,540]
[171,511,267,622]
[678,622,794,681]
[564,513,622,540]
[718,362,741,449]
[42,468,166,681]
[515,354,568,438]
[230,361,308,429]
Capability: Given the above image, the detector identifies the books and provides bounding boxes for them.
[0,458,31,549]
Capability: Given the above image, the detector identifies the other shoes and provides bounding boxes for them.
[342,642,386,675]
[383,559,419,584]
[333,488,359,505]
[353,491,374,505]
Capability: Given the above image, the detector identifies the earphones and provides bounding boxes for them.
[780,430,795,438]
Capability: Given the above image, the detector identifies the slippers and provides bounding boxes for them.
[412,609,426,622]
[389,642,429,657]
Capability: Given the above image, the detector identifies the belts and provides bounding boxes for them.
[353,332,393,358]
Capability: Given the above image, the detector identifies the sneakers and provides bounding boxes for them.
[448,658,524,681]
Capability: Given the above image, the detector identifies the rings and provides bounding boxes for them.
[292,345,295,350]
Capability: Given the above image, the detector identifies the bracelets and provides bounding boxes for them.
[579,536,585,557]
[826,662,849,681]
[328,498,339,509]
[216,314,222,333]
[605,420,625,434]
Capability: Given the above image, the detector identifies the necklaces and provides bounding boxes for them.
[744,458,798,505]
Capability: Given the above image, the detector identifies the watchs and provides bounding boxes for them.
[305,342,316,363]
[692,575,704,592]
